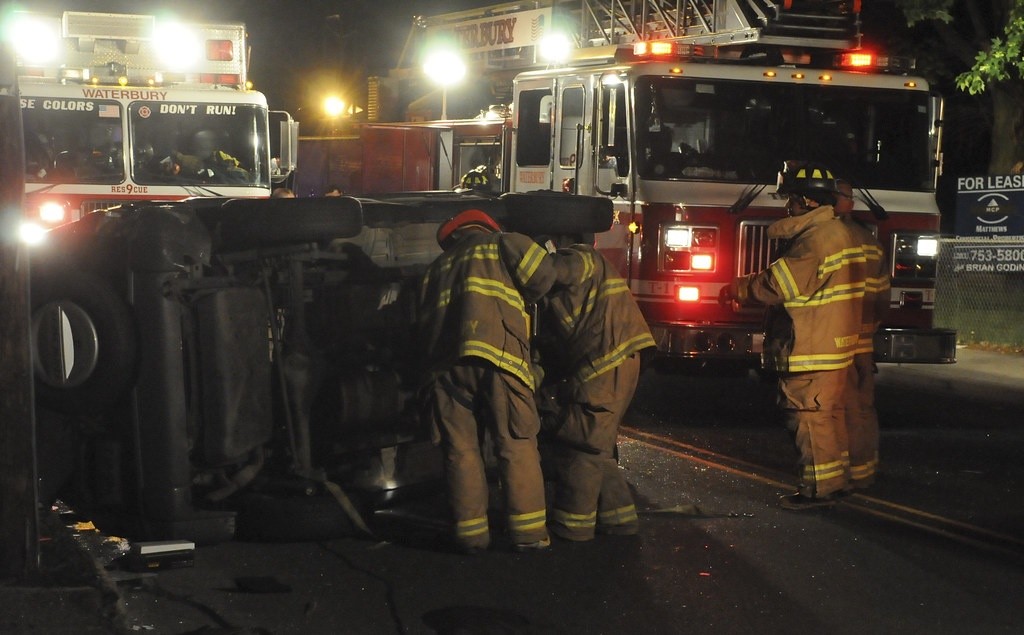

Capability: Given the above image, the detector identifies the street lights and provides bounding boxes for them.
[433,55,457,120]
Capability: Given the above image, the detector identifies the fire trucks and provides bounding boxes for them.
[0,9,298,233]
[293,0,959,364]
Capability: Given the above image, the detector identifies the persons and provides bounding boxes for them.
[271,187,295,198]
[410,208,656,551]
[717,164,891,508]
[323,185,342,196]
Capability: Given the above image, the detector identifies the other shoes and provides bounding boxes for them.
[596,524,639,536]
[779,491,836,510]
[513,533,551,554]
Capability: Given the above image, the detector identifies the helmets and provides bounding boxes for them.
[769,162,842,197]
[436,208,503,251]
